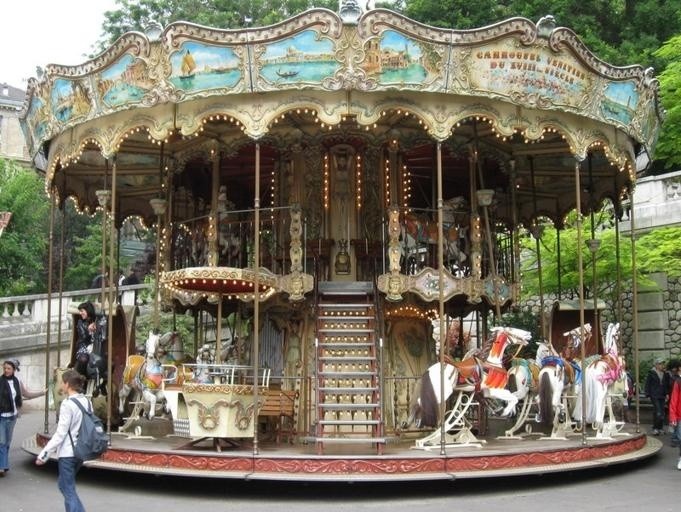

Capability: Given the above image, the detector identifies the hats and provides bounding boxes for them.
[655,358,665,365]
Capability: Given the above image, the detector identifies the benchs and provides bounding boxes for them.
[247,386,303,448]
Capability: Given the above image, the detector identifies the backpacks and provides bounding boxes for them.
[67,398,110,461]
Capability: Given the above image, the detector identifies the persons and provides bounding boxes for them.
[75,301,103,377]
[644,358,681,470]
[36,369,94,512]
[0,359,23,477]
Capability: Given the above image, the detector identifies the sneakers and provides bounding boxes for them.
[652,429,665,435]
[677,457,681,470]
